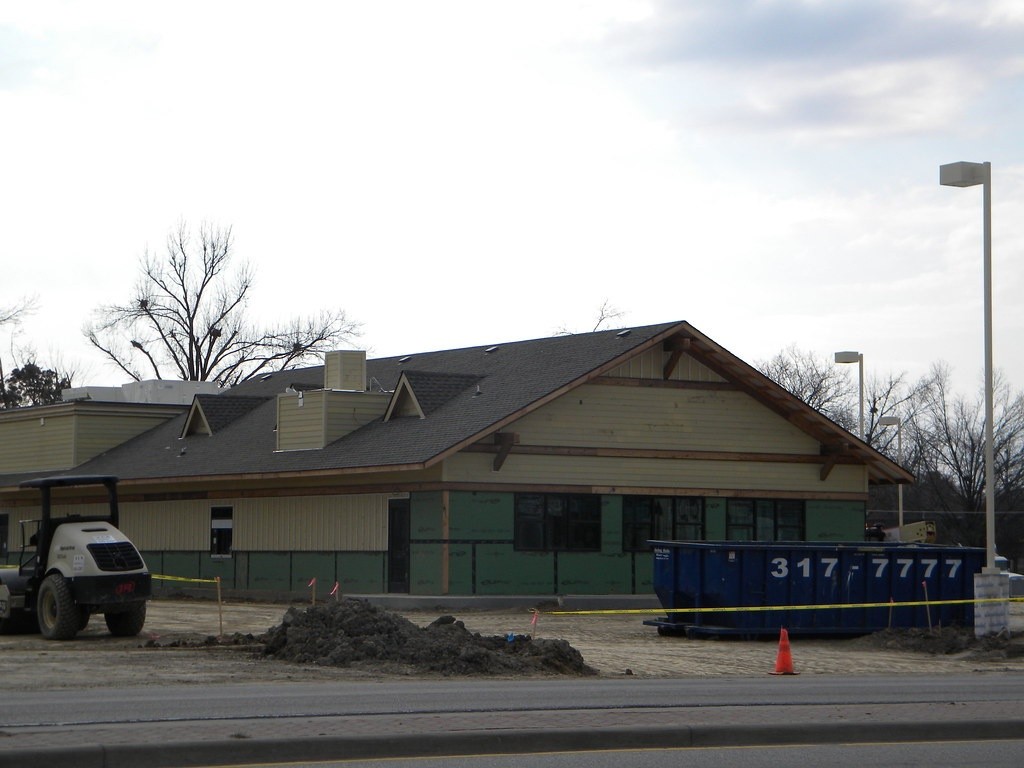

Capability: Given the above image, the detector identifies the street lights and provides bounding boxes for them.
[878,416,903,528]
[940,162,1003,573]
[835,351,864,440]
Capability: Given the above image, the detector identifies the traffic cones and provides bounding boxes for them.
[768,628,802,675]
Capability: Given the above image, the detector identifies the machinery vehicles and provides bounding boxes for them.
[0,474,152,642]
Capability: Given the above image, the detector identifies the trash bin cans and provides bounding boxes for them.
[642,539,988,641]
